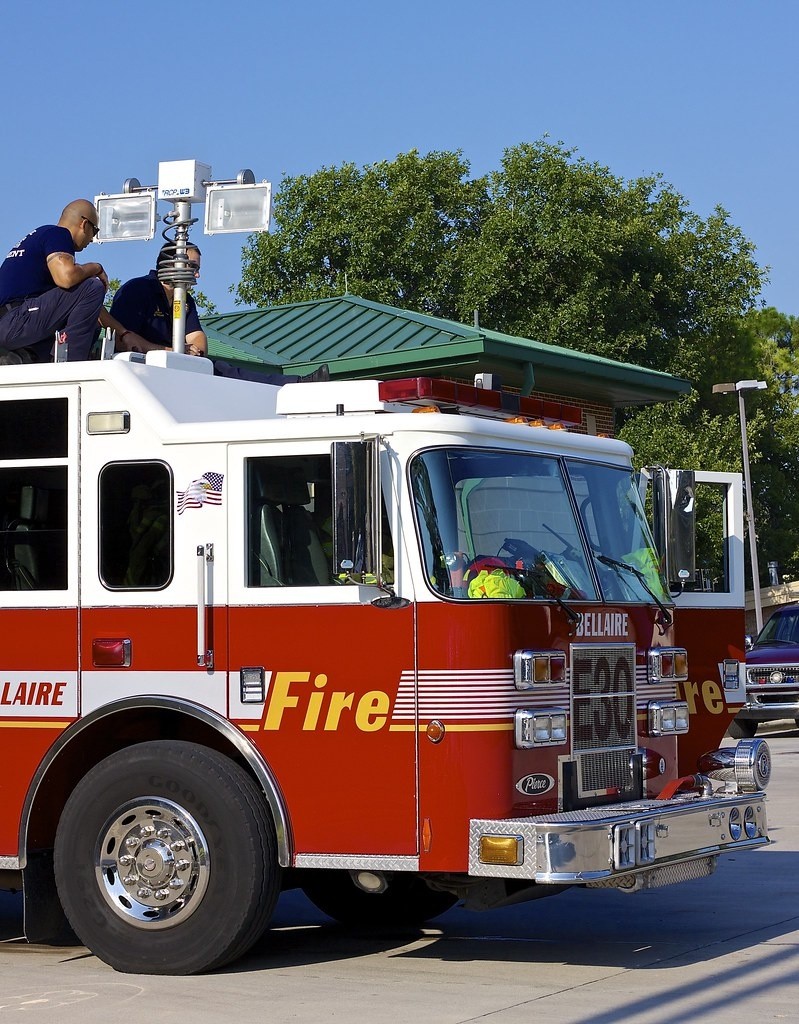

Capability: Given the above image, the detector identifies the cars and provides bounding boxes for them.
[728,602,799,738]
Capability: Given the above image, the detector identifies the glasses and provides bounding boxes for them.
[186,263,201,271]
[82,216,100,236]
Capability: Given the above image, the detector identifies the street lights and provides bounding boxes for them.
[709,379,769,634]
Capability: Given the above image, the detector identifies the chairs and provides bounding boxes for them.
[253,463,330,586]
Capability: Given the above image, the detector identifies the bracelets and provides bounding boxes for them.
[119,330,132,342]
[97,263,103,270]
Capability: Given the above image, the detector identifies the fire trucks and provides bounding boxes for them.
[0,158,779,978]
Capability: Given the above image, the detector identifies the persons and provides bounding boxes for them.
[0,197,143,365]
[109,241,329,386]
